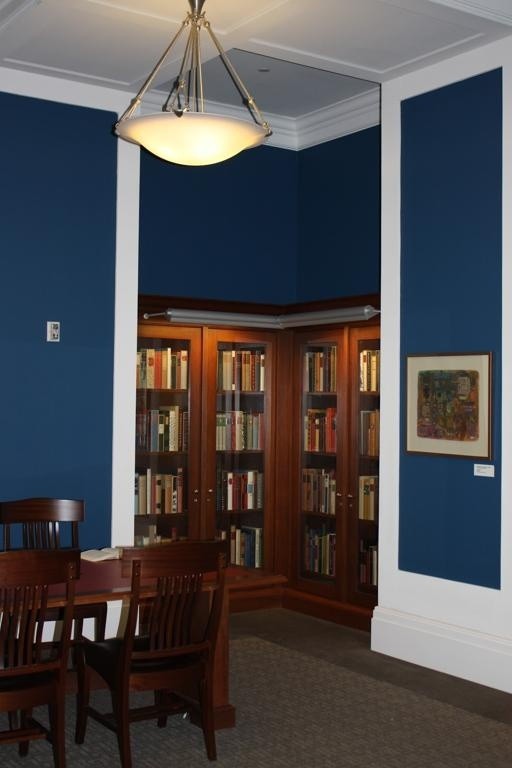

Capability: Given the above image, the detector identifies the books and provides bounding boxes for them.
[306,347,341,575]
[137,348,190,542]
[356,349,379,585]
[81,547,122,562]
[218,349,266,566]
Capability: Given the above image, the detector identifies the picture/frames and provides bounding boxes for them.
[405,350,493,459]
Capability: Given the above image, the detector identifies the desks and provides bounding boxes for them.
[2,538,288,731]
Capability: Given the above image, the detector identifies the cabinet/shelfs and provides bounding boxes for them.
[136,293,382,633]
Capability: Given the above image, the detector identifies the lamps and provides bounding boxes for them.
[110,2,274,170]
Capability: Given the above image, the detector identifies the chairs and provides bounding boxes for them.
[0,496,107,673]
[1,544,80,768]
[73,540,229,768]
